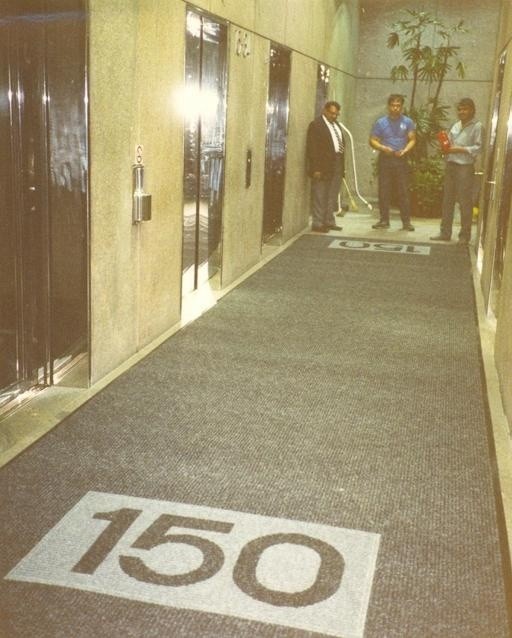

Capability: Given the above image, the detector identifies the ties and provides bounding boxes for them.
[329,120,345,155]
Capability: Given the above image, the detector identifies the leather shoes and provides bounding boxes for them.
[312,224,342,233]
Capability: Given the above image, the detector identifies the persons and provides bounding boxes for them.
[367,93,417,232]
[428,96,487,246]
[305,100,346,234]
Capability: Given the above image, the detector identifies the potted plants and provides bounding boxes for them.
[369,6,473,218]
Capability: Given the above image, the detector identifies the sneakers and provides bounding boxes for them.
[371,220,390,229]
[402,222,415,232]
[428,233,469,249]
